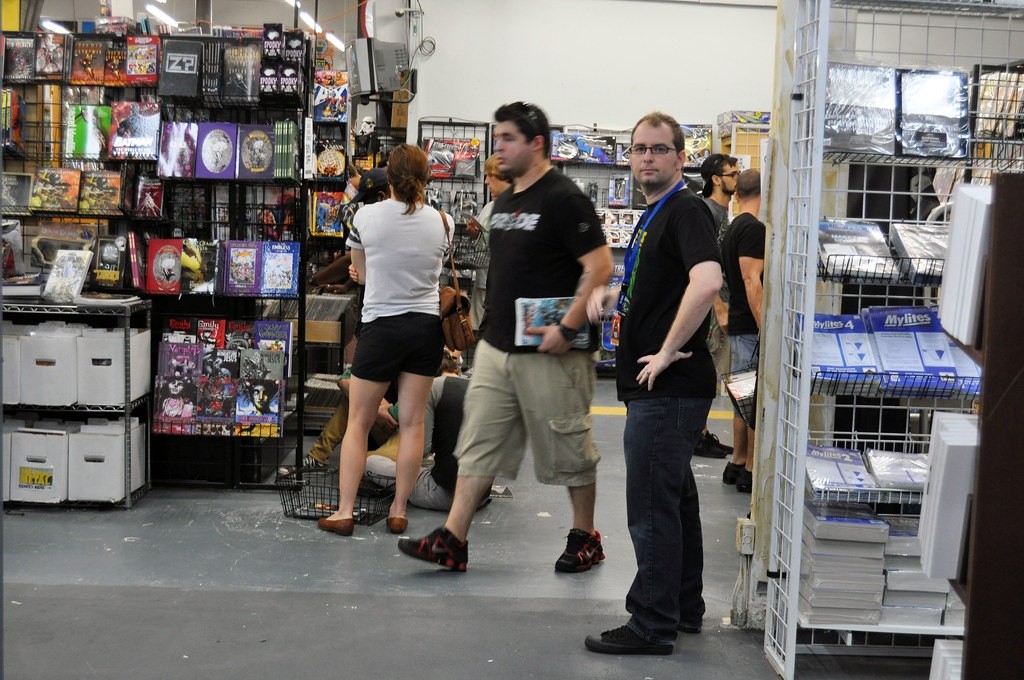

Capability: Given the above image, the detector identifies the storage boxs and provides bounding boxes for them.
[68,424,145,503]
[77,330,150,406]
[2,432,10,502]
[10,428,68,504]
[2,336,20,405]
[715,112,770,139]
[20,334,76,406]
[292,292,353,343]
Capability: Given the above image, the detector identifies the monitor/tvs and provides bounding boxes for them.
[344,37,408,98]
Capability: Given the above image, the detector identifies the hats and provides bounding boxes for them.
[350,168,387,203]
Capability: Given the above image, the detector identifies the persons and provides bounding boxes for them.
[691,153,766,494]
[397,103,614,573]
[318,142,455,536]
[282,165,494,513]
[586,112,725,655]
[467,152,512,332]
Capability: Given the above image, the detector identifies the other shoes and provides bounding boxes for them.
[386,517,408,534]
[735,468,753,494]
[723,460,747,485]
[318,516,354,536]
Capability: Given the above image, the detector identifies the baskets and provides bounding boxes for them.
[445,227,487,269]
[720,366,756,425]
[275,467,396,527]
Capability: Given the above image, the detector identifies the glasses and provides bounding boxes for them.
[716,171,741,179]
[631,145,680,154]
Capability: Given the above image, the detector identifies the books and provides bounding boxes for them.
[797,412,980,629]
[792,59,1024,402]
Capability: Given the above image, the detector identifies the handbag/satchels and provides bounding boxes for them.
[438,286,475,352]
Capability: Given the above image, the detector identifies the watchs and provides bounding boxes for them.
[559,324,577,341]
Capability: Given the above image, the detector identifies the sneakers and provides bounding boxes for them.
[554,528,605,573]
[398,526,468,573]
[704,431,735,454]
[278,455,328,478]
[677,617,701,634]
[584,620,673,655]
[692,433,727,458]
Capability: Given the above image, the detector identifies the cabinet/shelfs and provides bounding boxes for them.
[0,300,156,507]
[0,14,313,487]
[762,0,1023,679]
[415,117,713,373]
